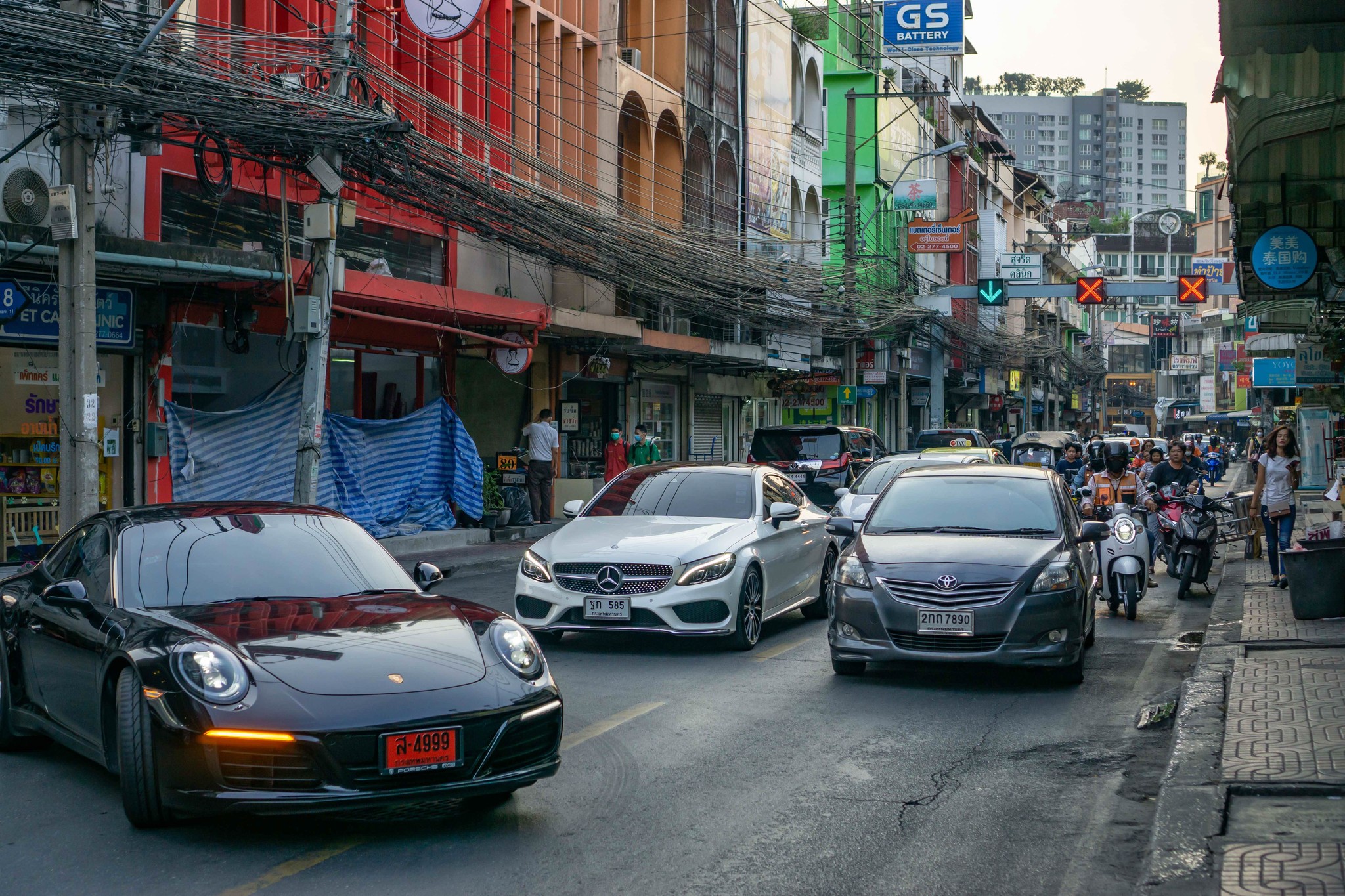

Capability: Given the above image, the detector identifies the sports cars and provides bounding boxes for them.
[0,499,566,834]
[513,460,841,653]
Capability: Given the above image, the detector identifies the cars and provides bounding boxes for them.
[828,453,992,553]
[990,439,1012,455]
[823,464,1112,688]
[922,447,1011,465]
[1011,423,1226,469]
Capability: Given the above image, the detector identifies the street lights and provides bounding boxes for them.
[1098,311,1149,434]
[1023,263,1106,433]
[843,141,970,426]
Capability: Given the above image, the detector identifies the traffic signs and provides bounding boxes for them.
[857,385,878,398]
[837,385,857,405]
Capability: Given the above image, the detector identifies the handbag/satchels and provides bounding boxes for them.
[1244,517,1262,560]
[1268,503,1291,517]
[1241,450,1247,457]
[1248,453,1259,462]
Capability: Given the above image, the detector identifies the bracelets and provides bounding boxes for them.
[1250,508,1257,509]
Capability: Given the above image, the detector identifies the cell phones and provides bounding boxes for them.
[1285,460,1301,468]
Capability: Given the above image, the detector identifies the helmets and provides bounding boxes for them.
[1087,440,1107,466]
[1185,441,1195,449]
[1185,435,1194,444]
[1149,446,1163,459]
[1216,436,1220,442]
[1073,441,1083,456]
[1229,438,1233,442]
[1195,434,1203,441]
[1209,435,1217,445]
[1105,441,1128,468]
[1130,438,1140,447]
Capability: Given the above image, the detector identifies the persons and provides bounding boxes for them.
[626,425,661,470]
[1165,434,1181,445]
[1055,434,1158,590]
[1222,371,1228,410]
[1226,437,1239,458]
[604,423,631,485]
[1138,446,1164,485]
[1001,432,1018,465]
[1182,433,1224,494]
[521,409,559,524]
[1245,432,1255,459]
[1248,426,1268,484]
[1145,440,1199,575]
[1250,426,1301,588]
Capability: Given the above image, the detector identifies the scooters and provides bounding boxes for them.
[1168,482,1235,601]
[1047,443,1238,565]
[1080,482,1159,621]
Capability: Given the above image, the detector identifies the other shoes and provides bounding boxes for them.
[1220,469,1226,476]
[1269,577,1288,589]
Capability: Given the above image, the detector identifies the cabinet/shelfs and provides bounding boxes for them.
[568,393,609,461]
[0,416,113,511]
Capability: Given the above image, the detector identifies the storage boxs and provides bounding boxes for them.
[1235,490,1266,533]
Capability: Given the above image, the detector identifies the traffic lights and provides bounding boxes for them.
[1179,274,1207,304]
[1077,277,1105,304]
[978,278,1005,304]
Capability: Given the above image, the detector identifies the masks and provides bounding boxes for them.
[635,433,645,441]
[611,431,622,441]
[1257,432,1264,437]
[1106,459,1124,473]
[1185,451,1192,456]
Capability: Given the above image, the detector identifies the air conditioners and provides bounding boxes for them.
[1038,380,1044,392]
[1182,385,1194,396]
[645,297,674,333]
[674,317,691,336]
[1039,358,1045,370]
[1109,269,1116,275]
[723,315,738,342]
[0,147,60,228]
[620,47,641,71]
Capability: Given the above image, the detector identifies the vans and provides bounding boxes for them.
[913,428,1004,453]
[747,423,901,513]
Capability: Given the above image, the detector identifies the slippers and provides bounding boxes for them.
[532,520,552,525]
[1148,569,1155,574]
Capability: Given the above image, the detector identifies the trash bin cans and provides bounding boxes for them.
[1298,537,1344,549]
[1278,548,1345,620]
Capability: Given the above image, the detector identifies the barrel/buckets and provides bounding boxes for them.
[13,449,20,463]
[20,449,29,463]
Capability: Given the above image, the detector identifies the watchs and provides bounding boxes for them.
[1292,477,1298,481]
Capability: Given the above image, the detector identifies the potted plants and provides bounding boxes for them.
[488,463,513,527]
[480,464,501,528]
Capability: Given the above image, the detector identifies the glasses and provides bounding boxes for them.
[1143,454,1150,459]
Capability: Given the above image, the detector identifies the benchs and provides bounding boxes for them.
[2,495,60,563]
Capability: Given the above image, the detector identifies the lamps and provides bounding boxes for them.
[812,285,845,298]
[808,283,831,293]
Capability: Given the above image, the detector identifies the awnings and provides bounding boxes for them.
[1183,412,1219,421]
[1207,413,1237,421]
[1227,409,1261,417]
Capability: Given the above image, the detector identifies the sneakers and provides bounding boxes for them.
[1096,575,1102,590]
[1147,577,1158,588]
